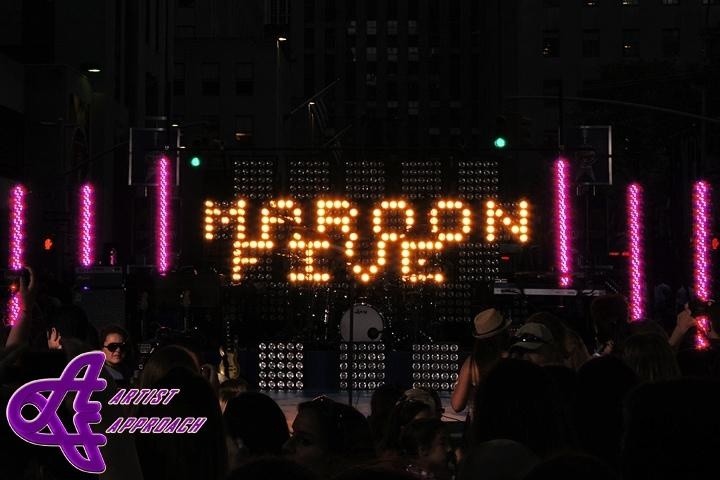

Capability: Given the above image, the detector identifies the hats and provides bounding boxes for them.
[510,322,552,349]
[473,308,512,340]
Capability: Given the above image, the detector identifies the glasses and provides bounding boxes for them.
[507,333,548,344]
[104,342,126,352]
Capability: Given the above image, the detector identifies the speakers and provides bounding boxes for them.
[128,127,180,187]
[558,124,612,187]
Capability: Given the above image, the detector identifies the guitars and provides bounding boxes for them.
[215,312,241,380]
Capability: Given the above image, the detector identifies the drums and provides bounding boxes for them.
[340,302,383,342]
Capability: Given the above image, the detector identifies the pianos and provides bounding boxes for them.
[494,288,606,296]
